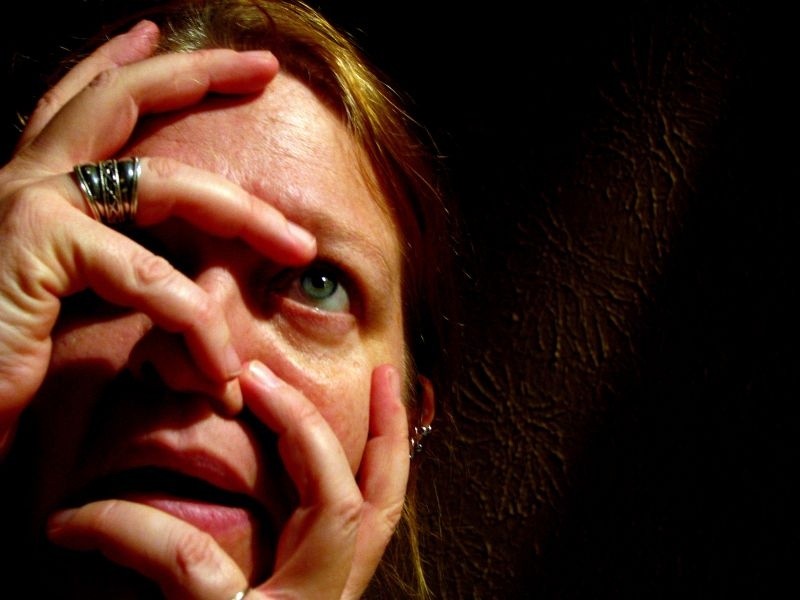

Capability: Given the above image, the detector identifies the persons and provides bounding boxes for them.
[0,0,459,600]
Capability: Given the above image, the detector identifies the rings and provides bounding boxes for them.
[73,156,142,228]
